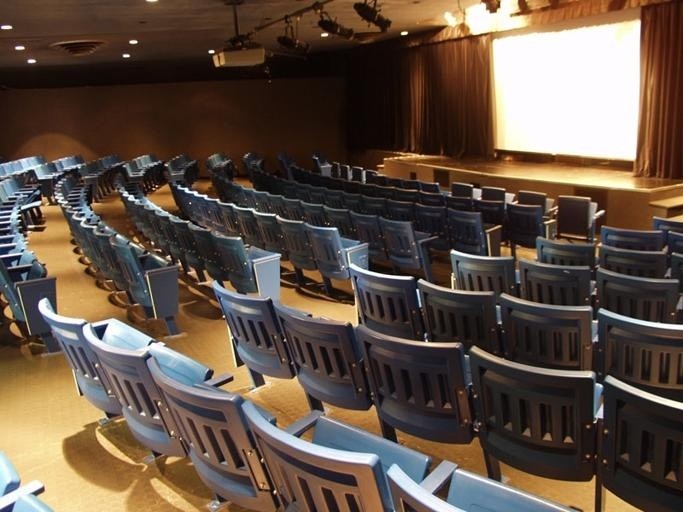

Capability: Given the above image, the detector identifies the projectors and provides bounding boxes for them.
[212,48,265,68]
